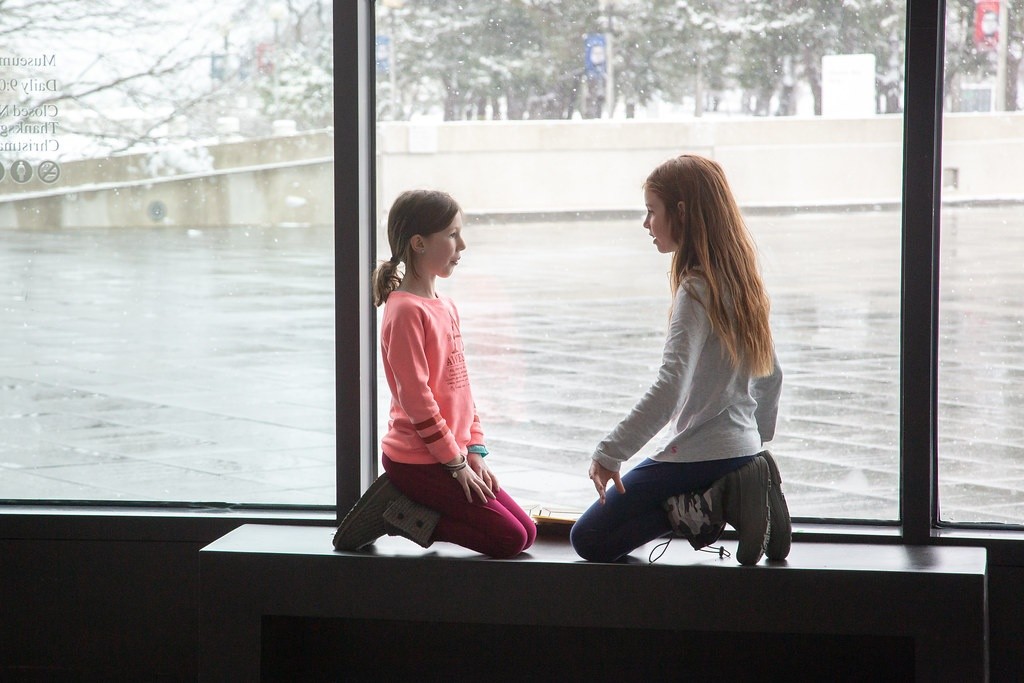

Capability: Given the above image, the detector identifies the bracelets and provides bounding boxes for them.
[445,454,467,478]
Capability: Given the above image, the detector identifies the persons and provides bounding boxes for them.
[570,154,792,566]
[332,191,536,559]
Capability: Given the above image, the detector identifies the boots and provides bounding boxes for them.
[331,471,441,551]
[663,456,772,567]
[758,450,792,561]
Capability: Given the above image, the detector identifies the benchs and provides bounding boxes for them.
[199,523,990,683]
